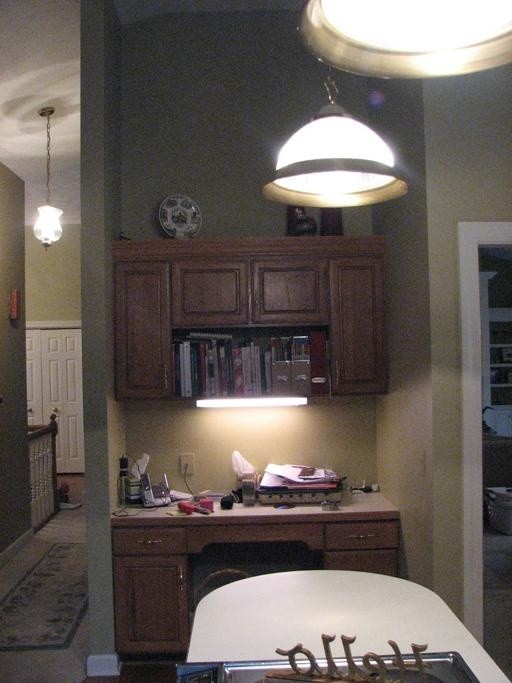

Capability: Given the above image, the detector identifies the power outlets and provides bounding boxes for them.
[179,451,197,476]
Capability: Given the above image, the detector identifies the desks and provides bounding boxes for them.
[111,489,407,667]
[179,559,511,681]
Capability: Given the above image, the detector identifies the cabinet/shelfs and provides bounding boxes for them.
[110,228,174,400]
[488,321,510,406]
[329,230,391,395]
[172,233,331,324]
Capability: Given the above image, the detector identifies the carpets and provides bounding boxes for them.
[1,540,90,652]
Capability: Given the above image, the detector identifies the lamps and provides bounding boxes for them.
[32,105,64,254]
[260,61,414,210]
[299,0,512,81]
[194,395,309,411]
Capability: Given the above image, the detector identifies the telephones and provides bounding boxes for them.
[140,473,171,508]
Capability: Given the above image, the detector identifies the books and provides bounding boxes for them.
[172,328,271,398]
[253,463,347,495]
